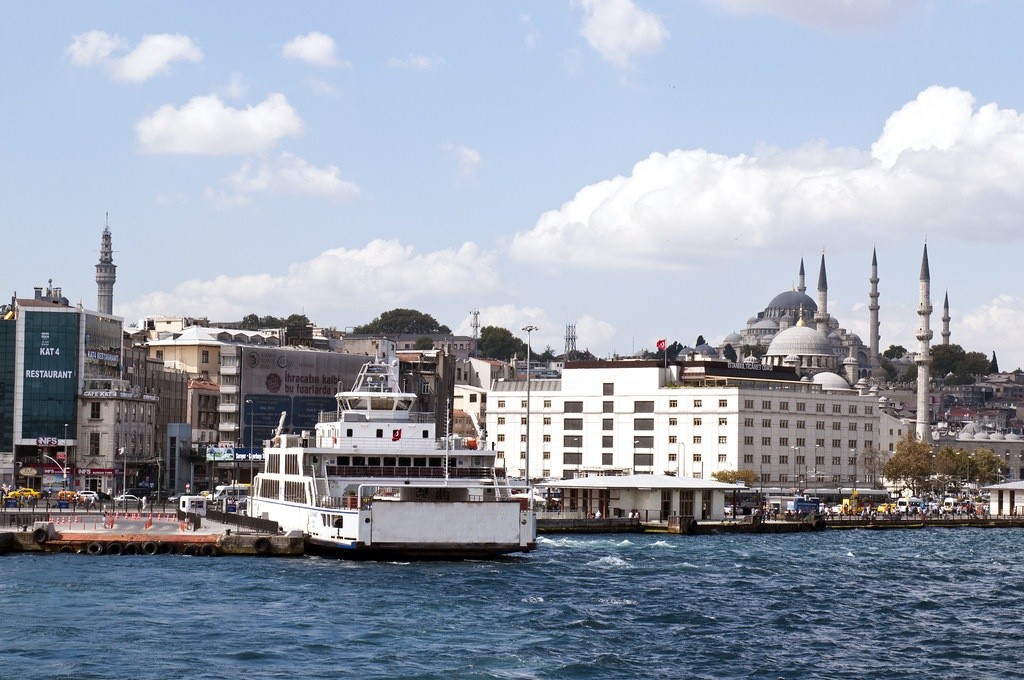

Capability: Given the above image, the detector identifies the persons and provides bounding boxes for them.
[19,489,112,510]
[751,507,803,523]
[587,509,641,518]
[824,503,976,521]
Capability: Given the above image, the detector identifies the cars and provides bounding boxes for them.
[943,496,975,512]
[206,484,250,502]
[8,488,42,497]
[76,488,100,501]
[896,497,922,513]
[114,494,142,502]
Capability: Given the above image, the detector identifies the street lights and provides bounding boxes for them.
[44,423,68,501]
[246,398,260,521]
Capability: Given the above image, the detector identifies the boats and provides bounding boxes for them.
[244,340,538,556]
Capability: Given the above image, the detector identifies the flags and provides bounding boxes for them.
[656,340,666,349]
[392,429,401,442]
